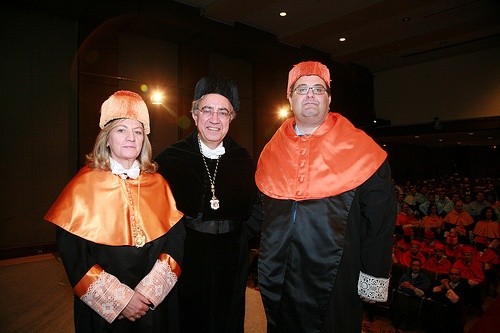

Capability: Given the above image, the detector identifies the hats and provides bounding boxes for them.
[287,60,331,98]
[194,73,240,112]
[99,90,150,135]
[475,237,489,245]
[461,245,474,254]
[446,233,458,244]
[411,240,422,247]
[434,243,446,251]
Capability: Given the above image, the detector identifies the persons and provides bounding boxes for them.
[389,178,500,333]
[156,75,256,333]
[43,90,186,333]
[255,61,397,333]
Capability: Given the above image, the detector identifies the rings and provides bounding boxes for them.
[136,315,139,319]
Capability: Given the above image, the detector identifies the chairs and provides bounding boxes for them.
[367,264,468,333]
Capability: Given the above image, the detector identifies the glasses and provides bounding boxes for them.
[291,83,330,95]
[197,108,232,120]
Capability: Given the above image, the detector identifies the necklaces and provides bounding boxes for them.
[117,174,146,248]
[198,133,220,209]
